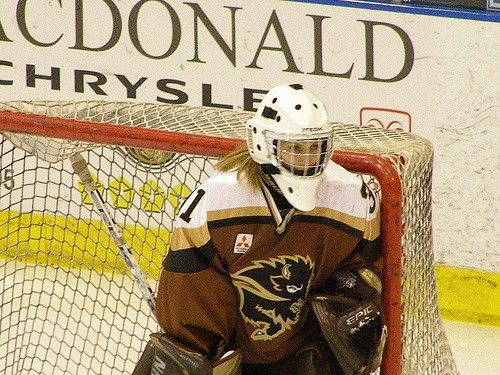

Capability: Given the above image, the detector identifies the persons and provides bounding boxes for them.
[155,82,389,375]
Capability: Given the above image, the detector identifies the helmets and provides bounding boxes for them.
[246,84,333,211]
